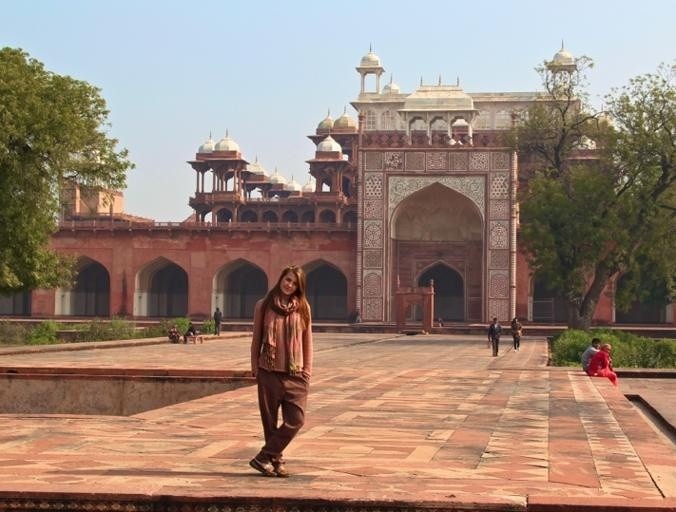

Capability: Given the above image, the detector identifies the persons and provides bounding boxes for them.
[213,307,223,335]
[249,265,311,477]
[588,343,618,385]
[511,318,523,351]
[168,324,180,343]
[488,317,502,356]
[581,338,601,371]
[183,321,194,343]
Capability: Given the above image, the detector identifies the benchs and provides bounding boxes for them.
[185,335,203,345]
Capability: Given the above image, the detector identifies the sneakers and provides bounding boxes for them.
[272,458,291,478]
[248,456,278,477]
